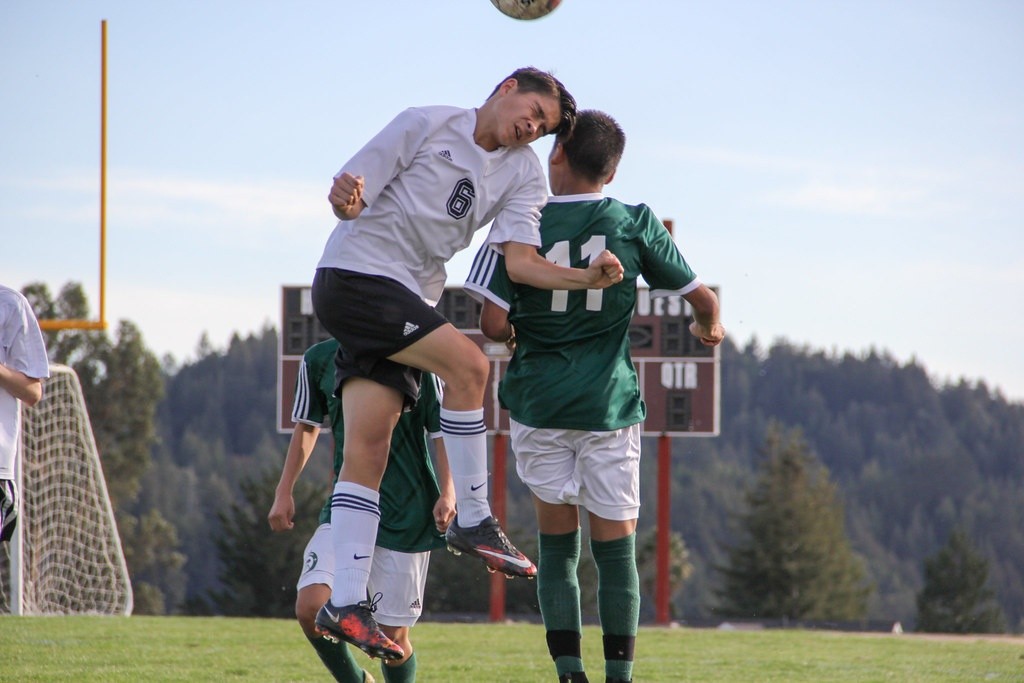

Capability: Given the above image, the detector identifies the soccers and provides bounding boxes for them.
[489,0,562,21]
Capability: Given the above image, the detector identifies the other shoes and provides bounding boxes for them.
[362,669,375,683]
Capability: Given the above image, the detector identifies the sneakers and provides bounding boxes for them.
[445,513,537,580]
[315,592,404,664]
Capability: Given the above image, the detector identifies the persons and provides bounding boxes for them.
[463,111,725,683]
[270,335,457,683]
[313,66,624,657]
[0,283,51,542]
